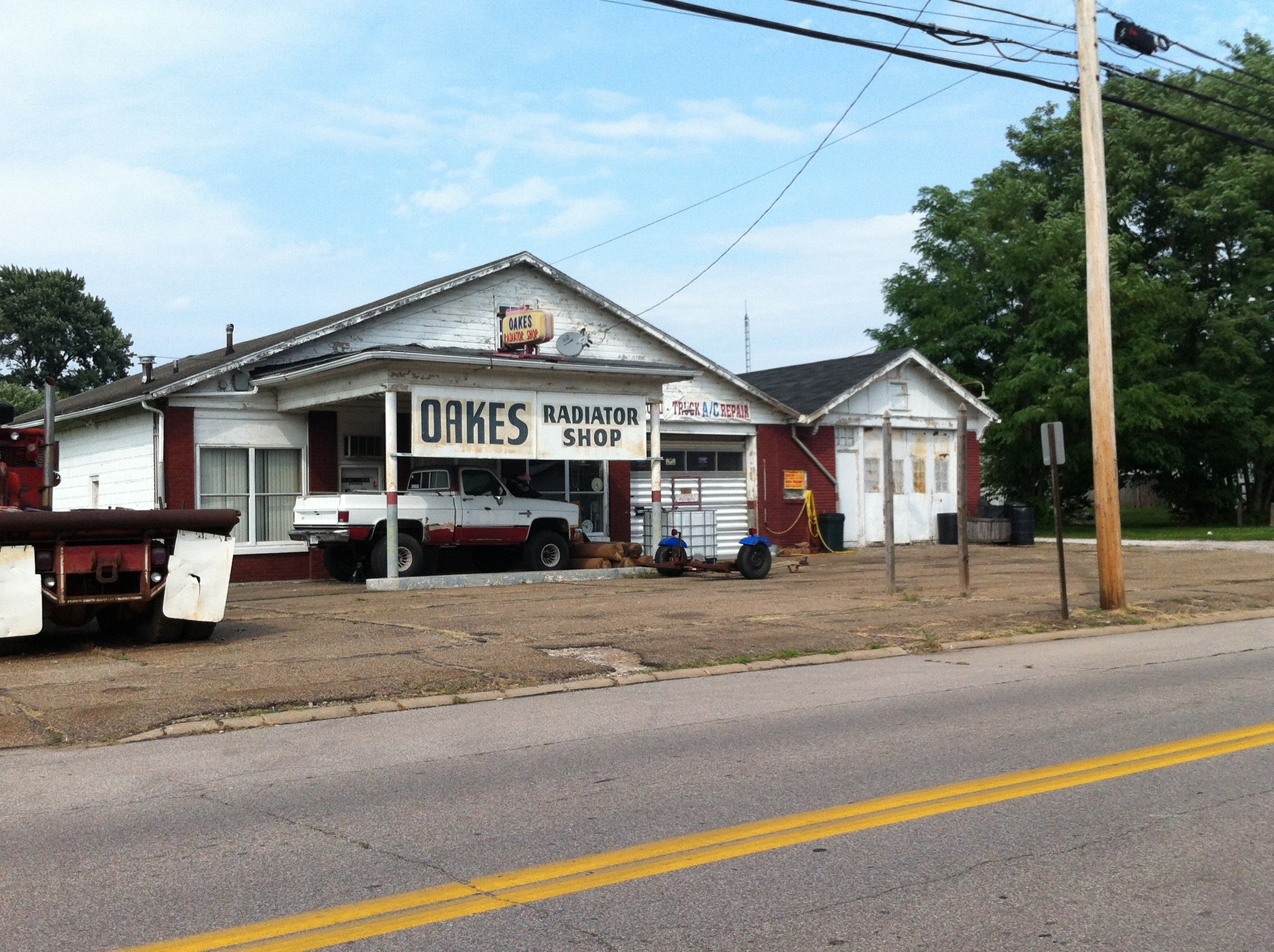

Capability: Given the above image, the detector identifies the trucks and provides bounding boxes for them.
[0,375,242,655]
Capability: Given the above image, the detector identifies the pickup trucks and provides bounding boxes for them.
[286,463,585,584]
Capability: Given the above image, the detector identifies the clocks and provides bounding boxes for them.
[592,478,603,491]
[582,520,593,532]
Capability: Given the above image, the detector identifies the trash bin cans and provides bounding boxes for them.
[818,513,846,553]
[1010,507,1034,545]
[937,513,957,544]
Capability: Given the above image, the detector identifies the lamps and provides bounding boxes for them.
[956,381,990,400]
[875,376,909,396]
